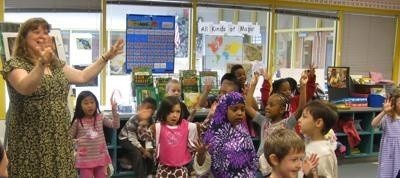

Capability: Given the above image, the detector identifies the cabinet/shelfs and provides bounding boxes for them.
[103,106,384,178]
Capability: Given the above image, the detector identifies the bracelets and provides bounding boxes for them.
[101,55,108,62]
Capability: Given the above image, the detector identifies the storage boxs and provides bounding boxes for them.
[368,94,385,107]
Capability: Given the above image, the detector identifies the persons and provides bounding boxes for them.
[117,63,339,178]
[71,89,121,178]
[370,87,399,178]
[2,16,125,178]
[0,141,9,178]
